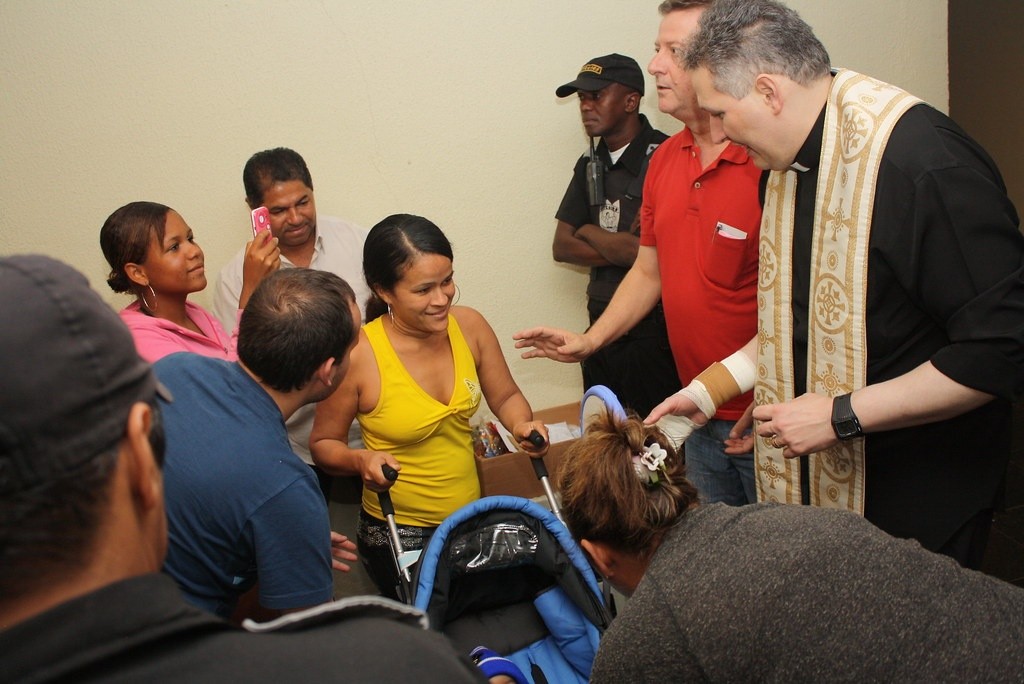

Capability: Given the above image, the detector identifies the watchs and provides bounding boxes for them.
[831,393,863,440]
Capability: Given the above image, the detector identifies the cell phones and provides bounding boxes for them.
[251,207,272,246]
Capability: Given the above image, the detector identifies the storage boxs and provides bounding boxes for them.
[473,399,583,499]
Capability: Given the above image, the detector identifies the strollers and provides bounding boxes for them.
[377,430,615,684]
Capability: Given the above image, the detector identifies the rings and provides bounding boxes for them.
[773,438,781,448]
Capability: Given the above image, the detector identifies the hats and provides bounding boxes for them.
[469,646,529,684]
[0,255,173,494]
[556,53,644,98]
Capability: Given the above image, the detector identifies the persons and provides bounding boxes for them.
[554,409,1024,684]
[310,213,550,607]
[0,252,487,684]
[212,146,372,501]
[513,0,767,508]
[466,644,529,684]
[99,201,282,371]
[642,0,1024,586]
[145,268,361,632]
[552,54,682,422]
